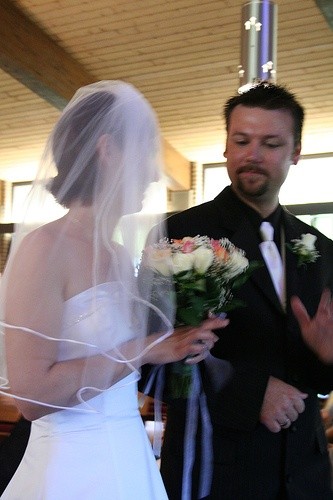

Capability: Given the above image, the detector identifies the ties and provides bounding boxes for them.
[260,221,288,314]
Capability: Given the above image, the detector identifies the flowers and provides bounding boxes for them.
[135,234,266,399]
[284,233,320,266]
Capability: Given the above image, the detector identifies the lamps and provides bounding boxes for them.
[235,0,278,95]
